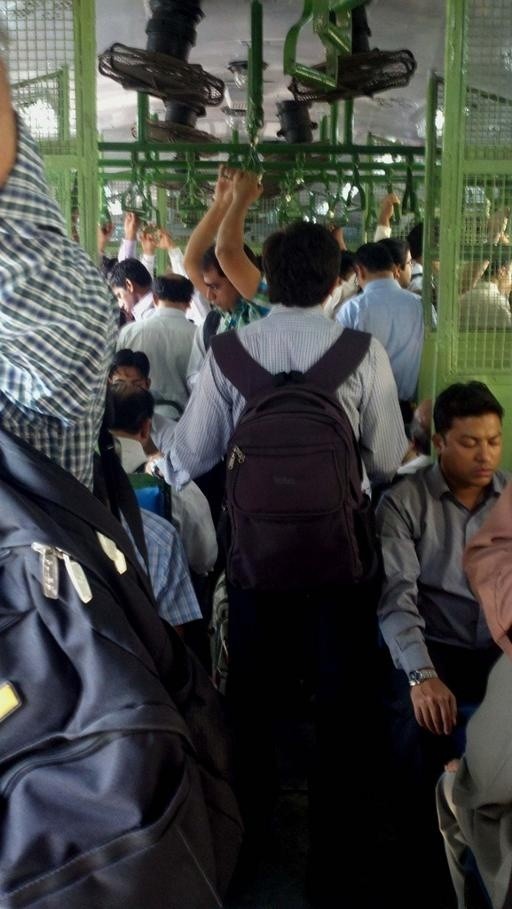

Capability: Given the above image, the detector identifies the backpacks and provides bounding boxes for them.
[1,421,244,903]
[210,326,381,590]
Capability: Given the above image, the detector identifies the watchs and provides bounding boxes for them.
[406,669,438,686]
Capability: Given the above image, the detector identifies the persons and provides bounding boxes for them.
[372,377,509,909]
[434,649,511,909]
[0,63,122,494]
[70,162,511,667]
[143,218,411,908]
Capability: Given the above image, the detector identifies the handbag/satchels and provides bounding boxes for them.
[377,579,503,706]
